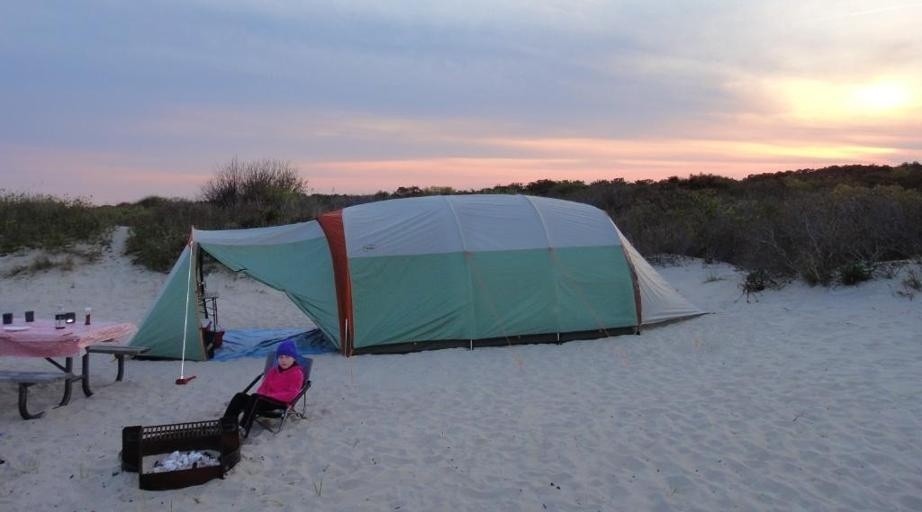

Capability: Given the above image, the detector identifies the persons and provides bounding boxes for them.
[218,339,304,439]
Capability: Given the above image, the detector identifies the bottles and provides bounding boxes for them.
[86,307,91,325]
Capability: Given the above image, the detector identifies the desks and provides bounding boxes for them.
[0,317,137,407]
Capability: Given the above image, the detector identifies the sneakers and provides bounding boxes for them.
[239,426,246,440]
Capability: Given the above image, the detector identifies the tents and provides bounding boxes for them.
[126,192,708,361]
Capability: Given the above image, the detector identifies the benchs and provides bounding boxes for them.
[85,345,152,382]
[1,369,73,418]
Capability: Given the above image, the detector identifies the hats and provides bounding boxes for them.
[276,339,298,359]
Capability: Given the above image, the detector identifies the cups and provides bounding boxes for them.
[25,311,34,322]
[55,312,75,329]
[3,313,12,324]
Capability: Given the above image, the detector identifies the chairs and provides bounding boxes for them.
[243,351,314,435]
